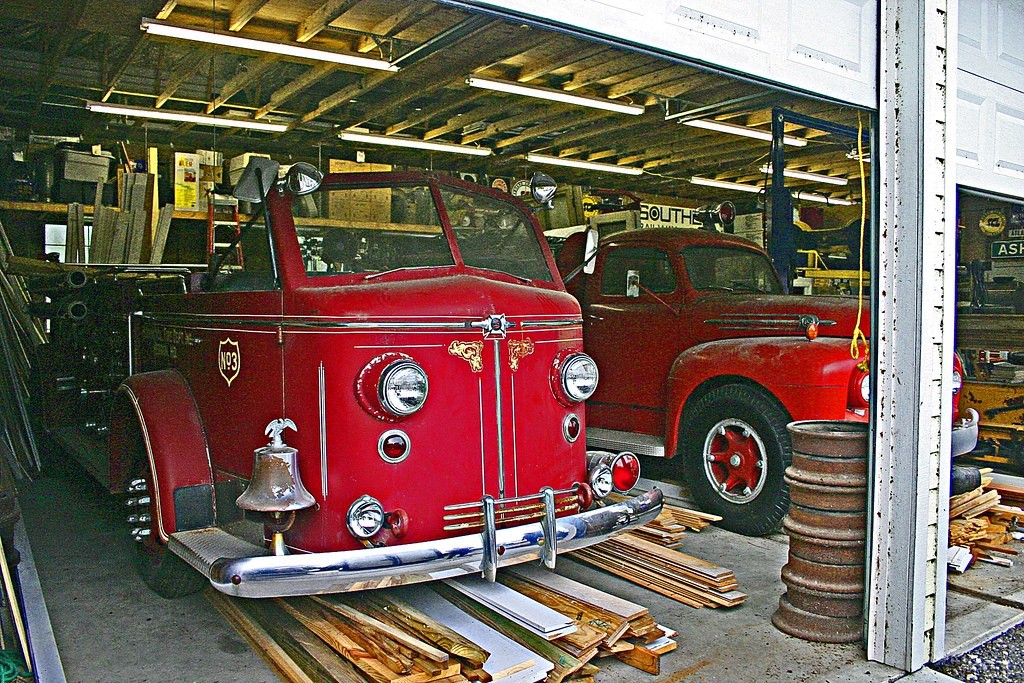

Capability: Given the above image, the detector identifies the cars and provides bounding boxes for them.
[547,200,981,539]
[40,157,663,598]
